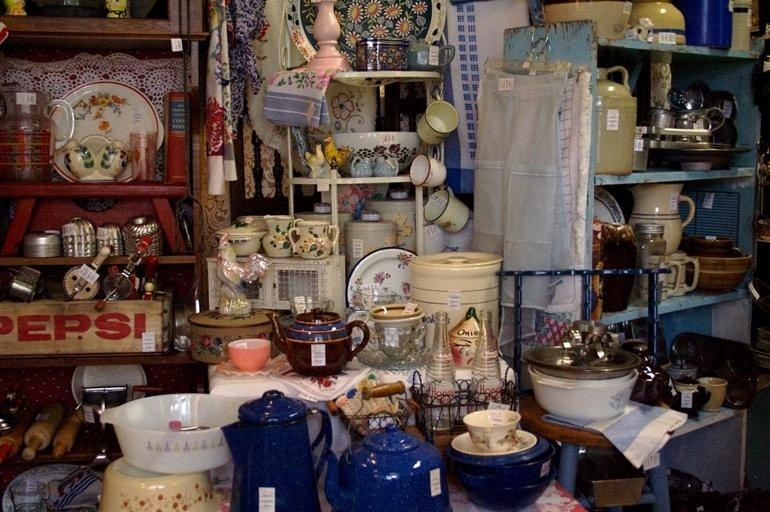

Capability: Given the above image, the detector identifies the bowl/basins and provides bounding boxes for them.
[529,365,642,423]
[61,215,96,260]
[685,255,752,293]
[648,31,688,45]
[94,222,124,256]
[226,338,271,371]
[215,232,267,257]
[370,296,425,319]
[444,437,557,510]
[99,456,221,512]
[462,409,522,450]
[120,213,165,257]
[101,392,255,475]
[540,0,633,40]
[635,0,685,31]
[367,311,426,328]
[72,365,147,419]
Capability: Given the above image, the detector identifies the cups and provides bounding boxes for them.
[372,323,426,360]
[417,97,463,148]
[667,252,702,297]
[639,260,681,302]
[696,376,730,412]
[406,152,449,191]
[128,130,159,183]
[418,187,471,235]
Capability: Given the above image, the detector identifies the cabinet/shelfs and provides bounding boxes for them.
[0,1,213,471]
[500,18,762,364]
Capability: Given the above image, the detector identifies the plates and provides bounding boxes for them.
[269,357,373,402]
[521,341,643,378]
[215,359,286,377]
[2,463,103,512]
[587,184,624,229]
[347,247,417,311]
[46,78,164,182]
[451,430,538,456]
[21,228,62,259]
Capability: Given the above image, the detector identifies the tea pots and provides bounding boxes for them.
[1,85,74,182]
[324,423,454,512]
[674,106,724,140]
[409,37,457,71]
[589,63,638,178]
[268,307,371,378]
[627,182,695,255]
[221,390,331,512]
